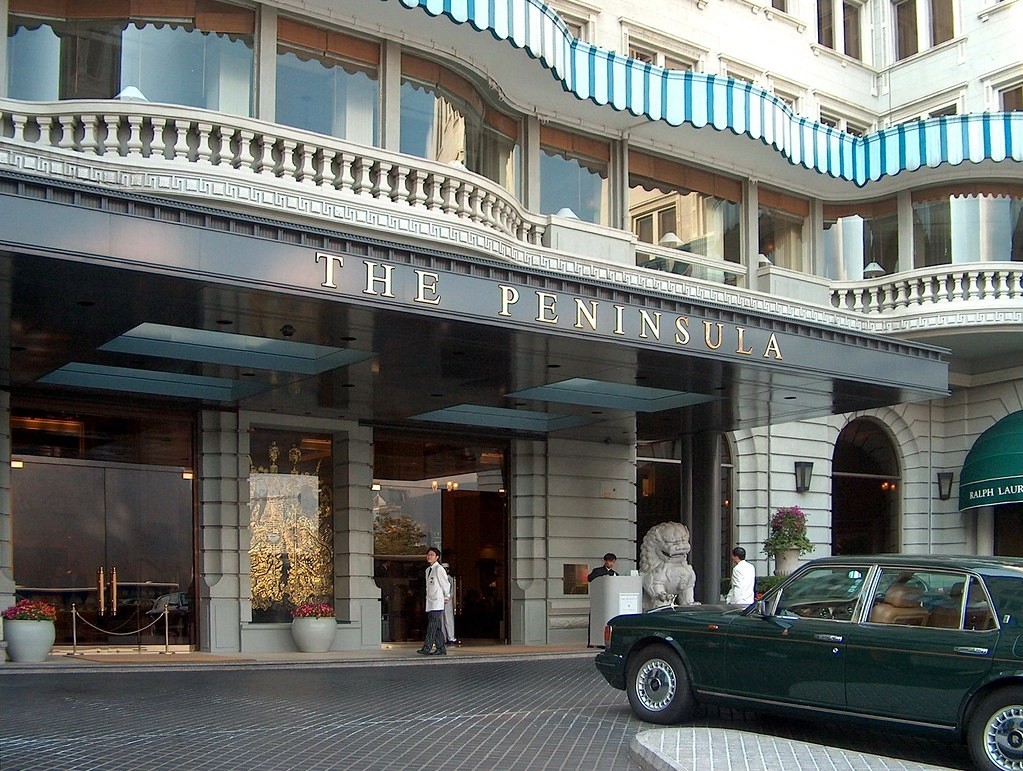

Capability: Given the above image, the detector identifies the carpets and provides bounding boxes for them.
[63,652,258,664]
[447,644,594,654]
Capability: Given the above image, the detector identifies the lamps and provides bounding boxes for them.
[863,263,886,278]
[758,254,773,268]
[113,86,148,102]
[448,160,468,171]
[937,472,953,500]
[659,232,684,273]
[556,208,581,220]
[795,461,814,491]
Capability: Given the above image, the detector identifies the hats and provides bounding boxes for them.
[443,562,451,568]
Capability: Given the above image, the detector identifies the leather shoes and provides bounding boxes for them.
[430,647,446,655]
[418,649,429,655]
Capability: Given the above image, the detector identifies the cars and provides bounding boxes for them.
[594,554,1023,771]
[119,592,190,636]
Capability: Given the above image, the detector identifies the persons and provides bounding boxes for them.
[441,563,462,644]
[417,547,451,655]
[587,553,620,649]
[374,553,396,642]
[60,559,89,644]
[726,547,755,608]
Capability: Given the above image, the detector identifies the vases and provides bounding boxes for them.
[2,618,56,662]
[773,545,801,576]
[291,617,337,653]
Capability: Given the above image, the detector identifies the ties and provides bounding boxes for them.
[428,567,432,577]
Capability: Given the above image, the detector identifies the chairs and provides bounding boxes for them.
[929,583,991,630]
[872,583,929,626]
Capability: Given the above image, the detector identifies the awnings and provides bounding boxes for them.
[959,409,1023,513]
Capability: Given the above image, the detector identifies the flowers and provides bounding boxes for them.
[761,505,816,562]
[1,599,57,622]
[288,597,336,621]
